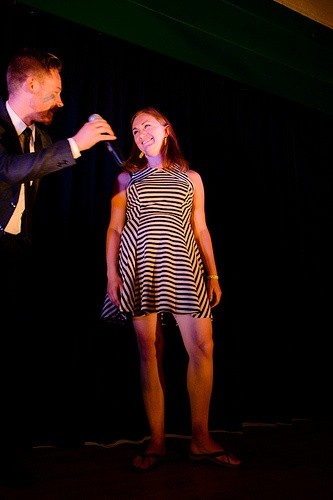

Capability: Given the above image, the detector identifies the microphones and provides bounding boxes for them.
[88,114,123,167]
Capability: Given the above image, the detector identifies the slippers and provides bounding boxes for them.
[193,445,242,469]
[22,128,35,211]
[134,447,164,469]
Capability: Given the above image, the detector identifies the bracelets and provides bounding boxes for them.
[206,276,218,280]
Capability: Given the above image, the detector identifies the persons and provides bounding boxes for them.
[1,46,115,433]
[98,108,242,470]
